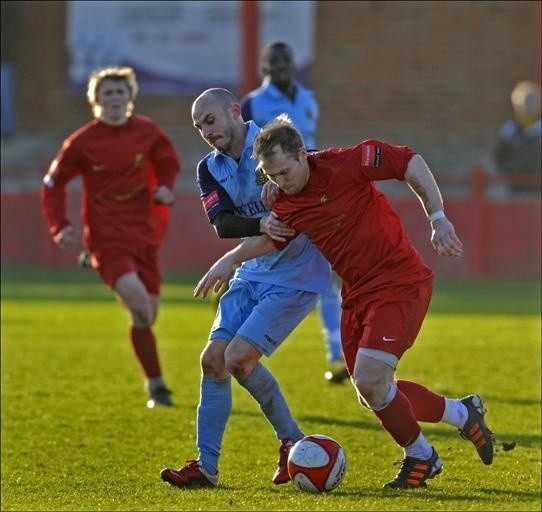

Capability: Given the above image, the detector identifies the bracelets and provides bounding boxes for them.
[428,210,445,224]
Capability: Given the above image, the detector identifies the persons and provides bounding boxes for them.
[40,66,180,406]
[193,114,493,488]
[159,87,332,489]
[493,80,542,192]
[238,41,350,383]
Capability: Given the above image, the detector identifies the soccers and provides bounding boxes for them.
[287,434,346,493]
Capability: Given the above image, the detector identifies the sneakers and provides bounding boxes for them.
[272,440,296,484]
[160,459,220,490]
[456,394,493,464]
[383,447,441,489]
